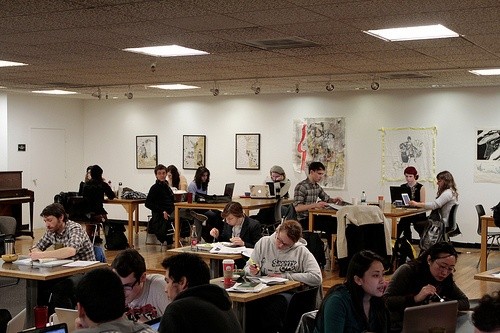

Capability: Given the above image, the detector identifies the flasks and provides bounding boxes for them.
[118,183,123,198]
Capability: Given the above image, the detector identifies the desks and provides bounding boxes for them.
[308,203,432,274]
[0,254,108,328]
[167,243,254,277]
[480,215,498,273]
[174,198,280,248]
[103,199,146,249]
[208,272,303,333]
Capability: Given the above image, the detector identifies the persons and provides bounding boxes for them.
[293,161,344,271]
[157,253,244,333]
[145,164,191,248]
[209,201,262,248]
[397,167,427,244]
[28,203,96,322]
[166,165,189,243]
[383,242,470,333]
[311,250,391,333]
[110,249,172,323]
[70,268,160,333]
[491,202,500,228]
[256,165,291,228]
[78,165,104,243]
[83,165,115,243]
[242,220,323,333]
[188,166,223,243]
[408,171,459,238]
[472,289,500,333]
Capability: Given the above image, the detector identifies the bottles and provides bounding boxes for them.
[191,226,197,250]
[361,191,367,205]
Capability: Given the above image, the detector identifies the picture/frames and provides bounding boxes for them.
[135,135,158,169]
[235,133,261,170]
[183,134,206,170]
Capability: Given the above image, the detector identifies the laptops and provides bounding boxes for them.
[16,323,68,333]
[390,186,412,205]
[144,317,161,333]
[250,185,276,199]
[224,183,235,199]
[402,300,459,333]
[55,307,78,333]
[401,193,420,209]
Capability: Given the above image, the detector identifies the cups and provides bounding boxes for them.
[187,192,192,203]
[352,198,357,204]
[393,200,402,207]
[222,259,234,287]
[4,239,15,254]
[378,196,383,209]
[49,313,58,326]
[245,192,250,195]
[33,306,48,328]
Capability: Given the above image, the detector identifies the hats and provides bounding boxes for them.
[270,166,285,175]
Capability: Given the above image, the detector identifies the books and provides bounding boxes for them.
[210,243,256,258]
[13,257,74,267]
[63,260,101,268]
[234,274,288,285]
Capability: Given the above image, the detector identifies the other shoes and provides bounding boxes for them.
[385,270,394,275]
[167,244,175,249]
[190,211,208,221]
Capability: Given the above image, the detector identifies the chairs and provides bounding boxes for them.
[66,192,103,247]
[285,286,319,333]
[475,204,500,270]
[329,205,394,278]
[0,216,20,288]
[428,204,462,257]
[259,198,282,236]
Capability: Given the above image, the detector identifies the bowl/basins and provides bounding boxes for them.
[232,269,244,280]
[2,254,17,262]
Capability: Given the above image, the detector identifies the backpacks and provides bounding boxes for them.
[395,238,417,269]
[420,219,443,250]
[103,219,129,251]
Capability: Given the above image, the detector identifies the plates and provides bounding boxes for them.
[458,312,468,319]
[212,242,233,246]
[197,244,213,250]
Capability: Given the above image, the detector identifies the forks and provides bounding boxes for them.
[428,284,444,302]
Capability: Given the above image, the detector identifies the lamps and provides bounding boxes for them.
[210,81,220,96]
[105,91,109,99]
[370,78,380,90]
[92,87,102,101]
[148,55,158,73]
[326,77,335,93]
[125,87,133,99]
[295,84,300,94]
[251,80,264,96]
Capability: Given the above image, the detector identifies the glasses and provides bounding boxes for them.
[277,231,295,248]
[435,260,456,273]
[123,279,139,290]
